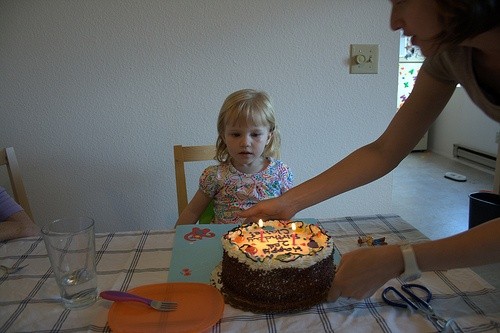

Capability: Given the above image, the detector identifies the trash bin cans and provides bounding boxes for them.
[468,191,500,231]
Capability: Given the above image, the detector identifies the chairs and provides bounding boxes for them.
[0,146,36,223]
[173,144,218,215]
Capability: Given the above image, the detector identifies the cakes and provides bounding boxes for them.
[220,219,337,309]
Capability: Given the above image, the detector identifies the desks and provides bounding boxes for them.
[0,214,500,333]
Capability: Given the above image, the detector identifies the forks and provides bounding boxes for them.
[99,290,177,312]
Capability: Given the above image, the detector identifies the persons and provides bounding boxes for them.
[174,89,294,228]
[236,1,499,303]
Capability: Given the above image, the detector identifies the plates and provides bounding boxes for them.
[107,282,225,333]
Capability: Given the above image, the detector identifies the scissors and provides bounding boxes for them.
[381,283,446,332]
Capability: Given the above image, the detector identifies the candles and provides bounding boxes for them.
[292,222,296,245]
[258,219,264,242]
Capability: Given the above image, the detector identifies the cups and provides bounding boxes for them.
[41,215,99,310]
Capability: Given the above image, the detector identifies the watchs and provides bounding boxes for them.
[399,243,422,283]
